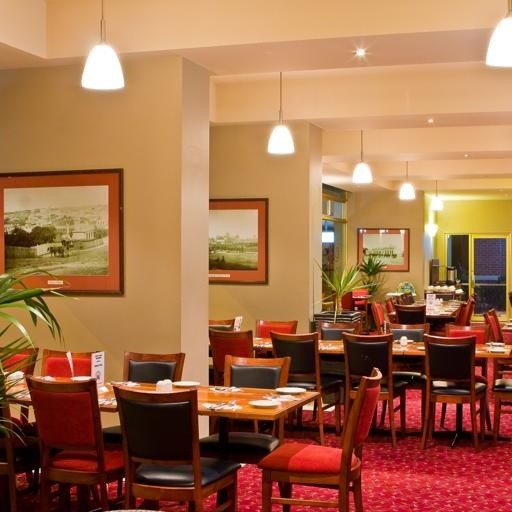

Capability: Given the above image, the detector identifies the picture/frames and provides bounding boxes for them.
[1,167,127,297]
[357,228,410,272]
[208,197,270,286]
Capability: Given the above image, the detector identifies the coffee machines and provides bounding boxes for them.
[446,266,457,283]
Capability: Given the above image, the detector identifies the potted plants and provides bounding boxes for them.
[312,257,385,336]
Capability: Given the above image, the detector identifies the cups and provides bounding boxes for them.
[426,285,463,294]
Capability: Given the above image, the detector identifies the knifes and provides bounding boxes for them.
[99,397,116,406]
[15,390,30,399]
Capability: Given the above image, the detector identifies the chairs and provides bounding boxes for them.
[0,290,511,512]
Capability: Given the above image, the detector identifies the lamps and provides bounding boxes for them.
[265,73,295,157]
[397,161,416,201]
[353,130,372,183]
[82,1,125,91]
[429,180,443,212]
[482,2,511,68]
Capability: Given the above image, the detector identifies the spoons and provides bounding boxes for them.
[207,399,237,411]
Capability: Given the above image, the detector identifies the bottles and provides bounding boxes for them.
[426,294,443,313]
[155,379,172,392]
[400,336,407,346]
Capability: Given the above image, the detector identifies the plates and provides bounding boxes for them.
[416,346,425,350]
[71,376,91,382]
[172,381,200,387]
[487,342,505,347]
[396,339,413,343]
[488,348,505,353]
[275,387,306,394]
[248,399,280,408]
